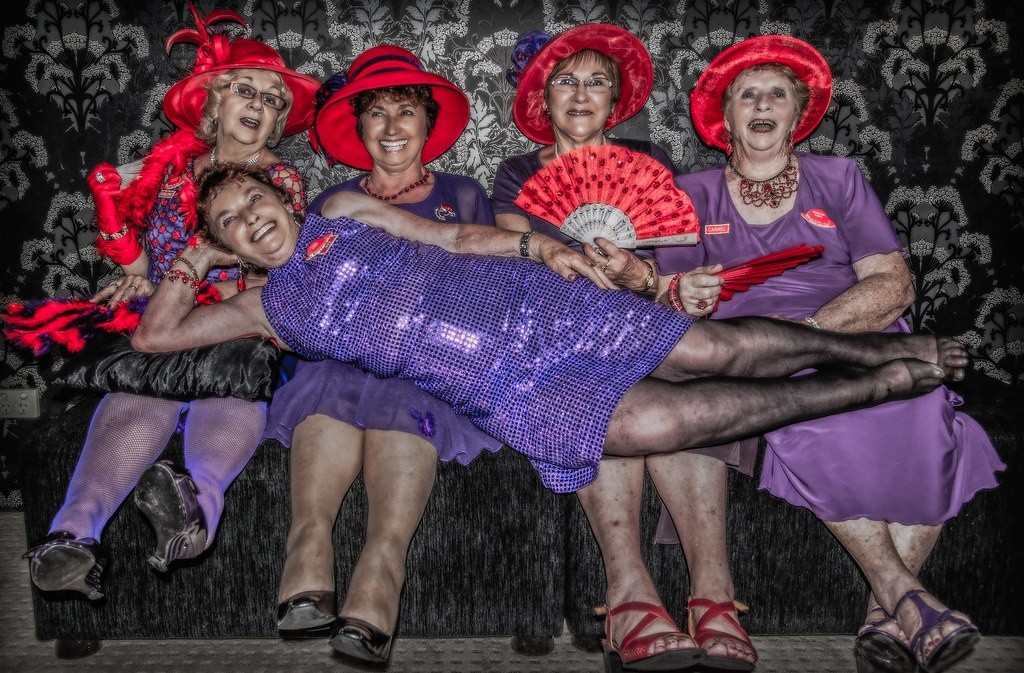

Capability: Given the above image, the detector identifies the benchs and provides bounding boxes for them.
[20,377,1024,661]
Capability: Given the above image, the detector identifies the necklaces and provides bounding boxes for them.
[726,153,800,209]
[365,166,430,202]
[555,135,606,158]
[211,146,261,166]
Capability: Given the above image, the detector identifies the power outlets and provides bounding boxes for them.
[0,389,40,419]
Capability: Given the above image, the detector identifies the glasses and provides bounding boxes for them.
[223,82,287,111]
[547,76,614,92]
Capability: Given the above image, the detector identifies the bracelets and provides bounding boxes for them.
[667,271,686,313]
[160,257,200,296]
[519,230,538,258]
[804,316,821,329]
[99,224,128,240]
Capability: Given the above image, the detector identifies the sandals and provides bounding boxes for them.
[892,589,981,673]
[856,607,928,673]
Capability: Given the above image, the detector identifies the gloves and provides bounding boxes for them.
[86,163,143,265]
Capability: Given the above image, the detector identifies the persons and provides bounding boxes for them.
[31,2,322,600]
[652,35,1008,673]
[276,45,495,663]
[130,159,969,493]
[494,23,758,673]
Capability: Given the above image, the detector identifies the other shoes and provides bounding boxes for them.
[275,591,338,631]
[329,616,392,663]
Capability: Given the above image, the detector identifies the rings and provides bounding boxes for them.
[696,300,707,310]
[589,264,596,267]
[94,170,106,184]
[113,283,119,290]
[128,286,138,292]
[601,260,610,272]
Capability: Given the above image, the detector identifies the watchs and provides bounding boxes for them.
[632,260,654,294]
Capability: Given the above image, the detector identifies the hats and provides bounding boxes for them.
[309,45,470,171]
[163,0,323,137]
[690,35,833,150]
[506,23,653,145]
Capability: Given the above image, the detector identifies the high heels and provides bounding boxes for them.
[21,530,106,600]
[685,595,757,670]
[133,460,207,573]
[593,601,707,673]
